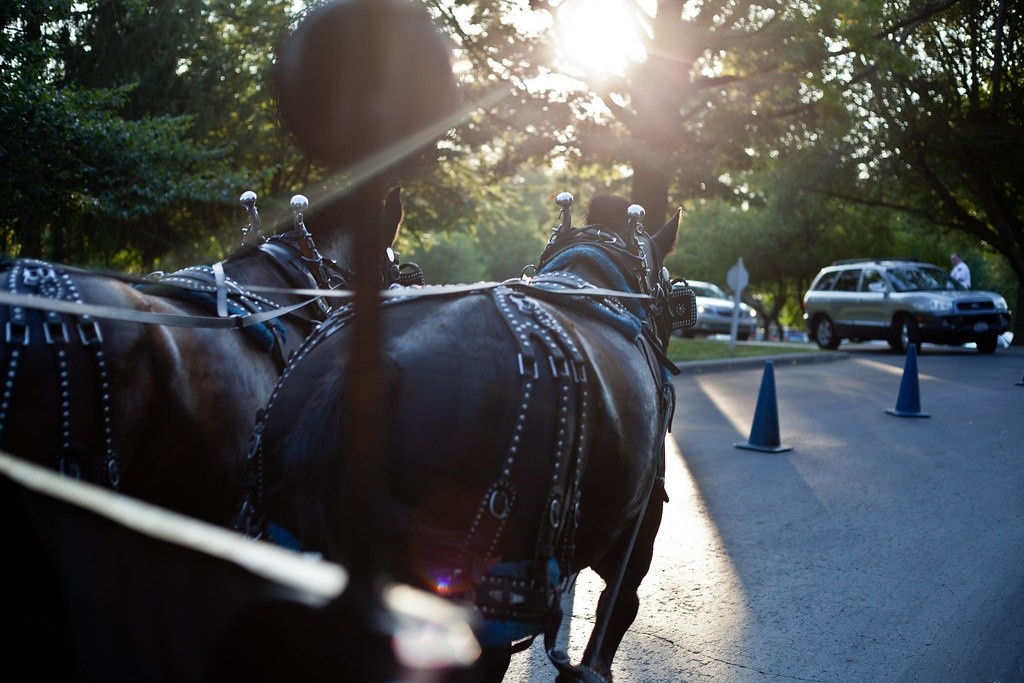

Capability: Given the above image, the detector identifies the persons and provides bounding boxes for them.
[944,254,971,289]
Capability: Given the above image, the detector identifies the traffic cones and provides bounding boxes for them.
[732,360,794,454]
[885,343,932,419]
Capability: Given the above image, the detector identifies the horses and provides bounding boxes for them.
[0,185,684,683]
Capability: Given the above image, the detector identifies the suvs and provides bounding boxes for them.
[674,279,762,341]
[799,258,1014,358]
